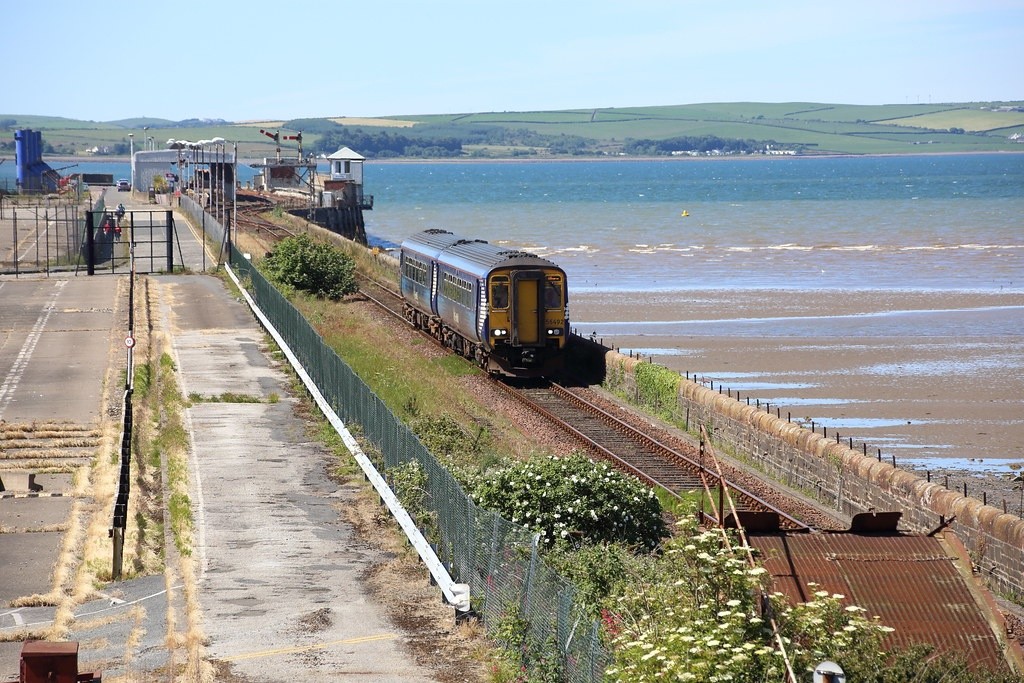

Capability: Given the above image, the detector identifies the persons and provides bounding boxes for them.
[102,204,126,241]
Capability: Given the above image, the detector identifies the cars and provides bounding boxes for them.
[118,181,130,192]
[82,182,89,192]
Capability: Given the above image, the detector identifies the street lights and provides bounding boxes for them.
[143,126,149,151]
[148,137,152,152]
[128,134,135,197]
[152,136,156,150]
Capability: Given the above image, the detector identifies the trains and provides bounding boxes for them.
[399,228,570,380]
